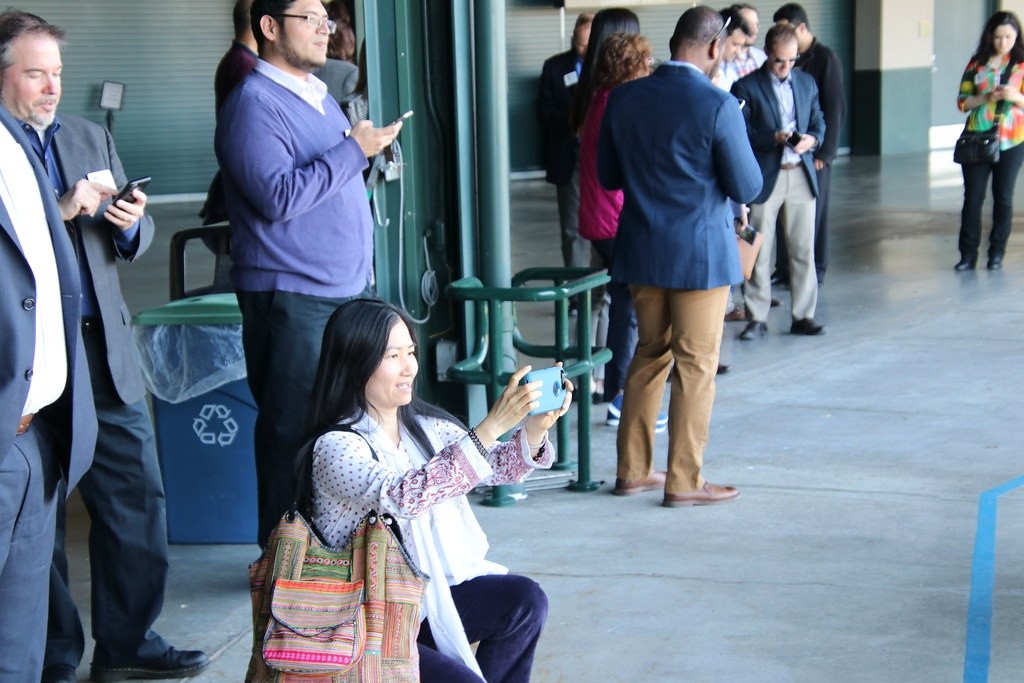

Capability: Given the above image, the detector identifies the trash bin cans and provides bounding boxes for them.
[131,292,257,545]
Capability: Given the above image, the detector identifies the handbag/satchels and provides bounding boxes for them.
[245,426,431,683]
[954,124,1000,165]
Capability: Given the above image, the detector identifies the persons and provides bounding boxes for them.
[306,300,572,683]
[0,11,209,683]
[542,4,848,431]
[227,0,406,556]
[595,6,764,507]
[214,0,360,281]
[953,12,1024,271]
[0,106,98,683]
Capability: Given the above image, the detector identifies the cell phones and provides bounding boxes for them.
[786,130,800,149]
[107,176,151,218]
[734,218,756,245]
[995,85,1007,92]
[524,367,566,415]
[738,99,745,108]
[385,110,414,127]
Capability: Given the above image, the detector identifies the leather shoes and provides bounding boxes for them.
[663,481,740,508]
[612,470,666,496]
[42,662,77,683]
[89,645,210,683]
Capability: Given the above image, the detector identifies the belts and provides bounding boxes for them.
[780,161,803,170]
[16,412,34,434]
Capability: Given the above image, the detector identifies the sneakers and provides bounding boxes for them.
[607,393,668,425]
[607,410,666,433]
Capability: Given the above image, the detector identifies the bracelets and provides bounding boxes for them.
[530,441,545,448]
[468,428,489,457]
[533,443,545,460]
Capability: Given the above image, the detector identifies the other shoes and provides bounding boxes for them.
[987,255,1000,269]
[791,318,828,335]
[572,388,579,403]
[740,321,767,339]
[717,364,730,374]
[770,268,782,286]
[770,297,784,307]
[954,258,976,271]
[816,267,824,284]
[724,305,746,321]
[592,392,604,404]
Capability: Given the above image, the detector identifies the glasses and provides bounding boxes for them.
[770,48,800,62]
[269,12,337,35]
